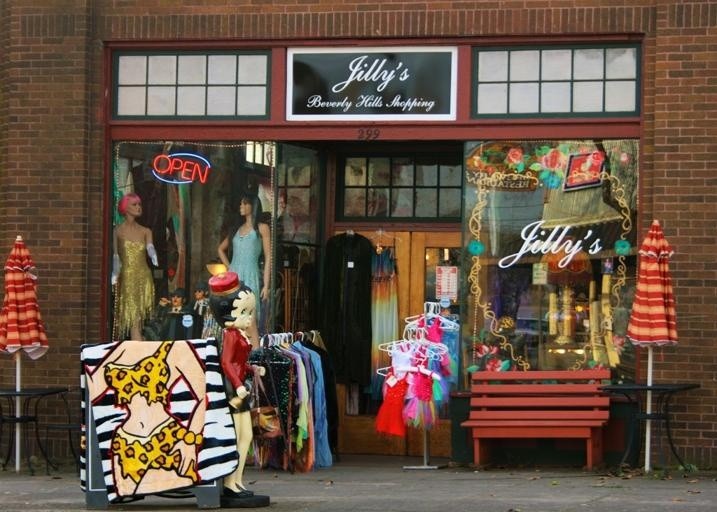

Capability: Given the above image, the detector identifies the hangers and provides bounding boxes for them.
[366,226,401,243]
[344,225,354,236]
[374,301,459,381]
[257,330,320,350]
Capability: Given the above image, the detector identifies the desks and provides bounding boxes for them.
[595,381,700,471]
[0,384,77,473]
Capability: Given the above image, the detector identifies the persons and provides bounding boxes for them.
[156,287,188,338]
[219,194,270,335]
[210,272,267,498]
[86,339,207,496]
[110,194,160,341]
[190,280,208,338]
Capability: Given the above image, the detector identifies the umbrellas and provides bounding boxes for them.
[0,235,49,472]
[625,219,679,473]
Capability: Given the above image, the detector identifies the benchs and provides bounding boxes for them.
[459,368,614,470]
